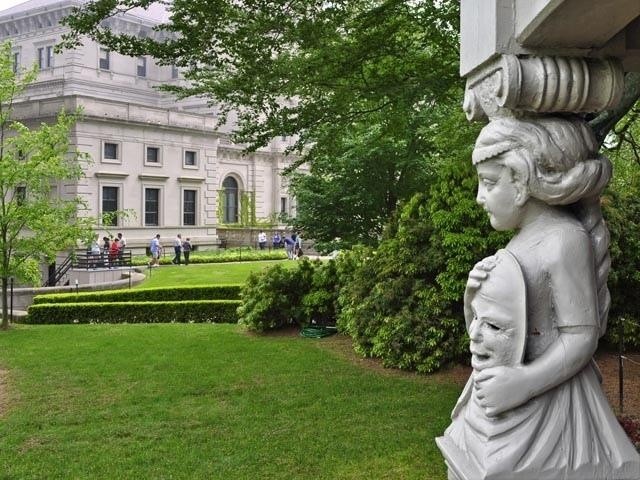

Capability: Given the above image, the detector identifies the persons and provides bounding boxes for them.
[183,238,192,266]
[171,233,182,264]
[435,116,640,480]
[86,232,129,267]
[148,234,162,267]
[272,230,304,261]
[258,229,266,249]
